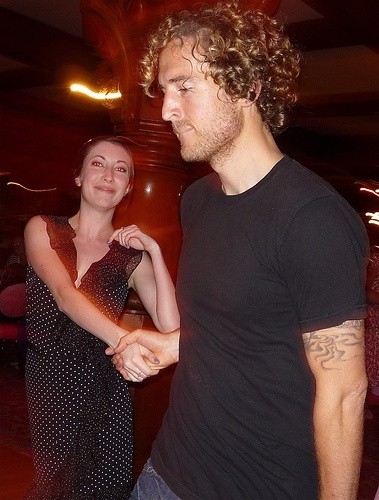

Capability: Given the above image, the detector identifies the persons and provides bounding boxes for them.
[21,135,179,500]
[104,0,368,500]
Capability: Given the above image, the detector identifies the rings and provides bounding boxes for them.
[137,371,142,375]
[121,226,124,229]
